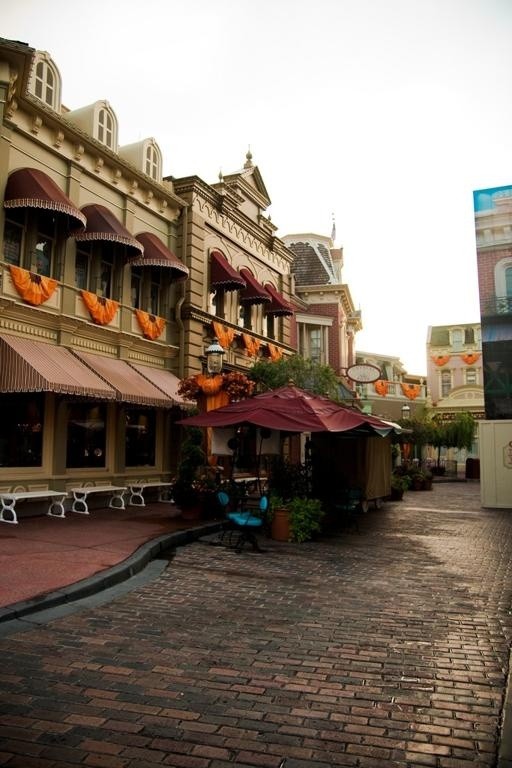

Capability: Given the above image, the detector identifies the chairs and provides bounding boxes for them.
[233,496,269,553]
[217,492,252,545]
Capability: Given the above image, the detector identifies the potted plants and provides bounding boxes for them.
[391,475,408,501]
[266,494,321,544]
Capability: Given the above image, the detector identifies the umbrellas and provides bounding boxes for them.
[172,377,398,473]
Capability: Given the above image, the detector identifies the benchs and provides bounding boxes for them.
[192,477,268,496]
[0,482,177,524]
